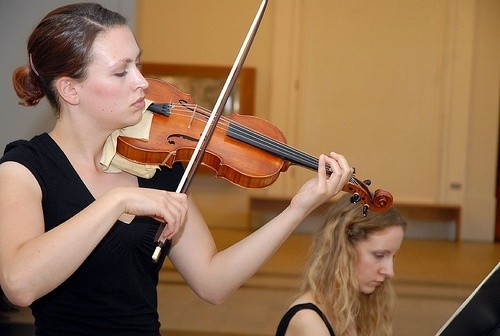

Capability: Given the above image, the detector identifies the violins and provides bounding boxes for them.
[108,76,392,218]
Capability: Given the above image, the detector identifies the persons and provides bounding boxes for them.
[1,1,353,336]
[275,190,408,336]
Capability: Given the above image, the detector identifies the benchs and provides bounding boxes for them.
[248,194,461,244]
